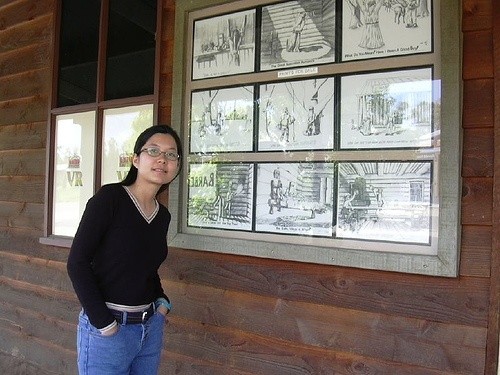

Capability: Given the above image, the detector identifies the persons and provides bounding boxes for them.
[67,124,184,375]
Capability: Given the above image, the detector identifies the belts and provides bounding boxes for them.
[108,304,156,324]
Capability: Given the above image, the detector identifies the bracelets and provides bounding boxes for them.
[155,299,171,313]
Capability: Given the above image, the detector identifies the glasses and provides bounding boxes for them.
[140,147,180,161]
